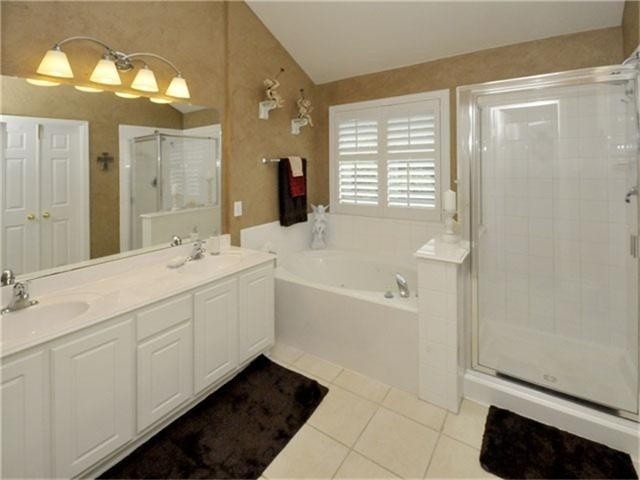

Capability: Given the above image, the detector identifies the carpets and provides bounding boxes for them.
[96,353,330,478]
[479,405,639,480]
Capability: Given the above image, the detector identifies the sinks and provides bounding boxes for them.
[0,291,103,343]
[169,250,237,273]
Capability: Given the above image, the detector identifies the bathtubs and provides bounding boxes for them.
[275,245,419,395]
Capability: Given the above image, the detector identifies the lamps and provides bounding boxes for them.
[39,35,193,100]
[23,75,176,104]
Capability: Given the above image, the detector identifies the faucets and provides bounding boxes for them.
[394,272,408,299]
[168,235,181,247]
[8,281,30,311]
[191,242,206,259]
[1,269,15,286]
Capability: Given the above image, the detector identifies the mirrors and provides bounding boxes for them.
[0,80,211,270]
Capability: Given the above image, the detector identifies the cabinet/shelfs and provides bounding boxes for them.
[0,241,279,479]
